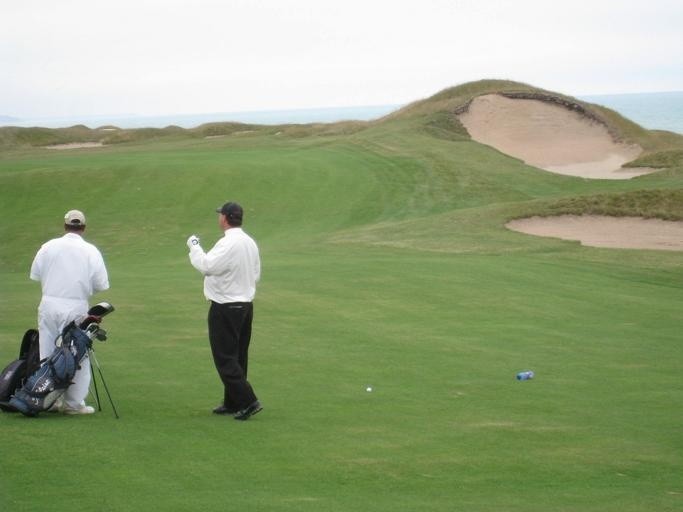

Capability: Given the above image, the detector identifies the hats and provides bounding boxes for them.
[65,210,86,226]
[216,202,243,219]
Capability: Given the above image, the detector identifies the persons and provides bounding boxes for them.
[27,210,110,415]
[184,200,264,419]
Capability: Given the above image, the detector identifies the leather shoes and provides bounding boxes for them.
[213,405,241,414]
[235,401,263,419]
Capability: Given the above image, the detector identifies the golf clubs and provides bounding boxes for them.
[85,322,106,342]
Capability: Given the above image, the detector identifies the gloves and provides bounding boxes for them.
[187,236,200,248]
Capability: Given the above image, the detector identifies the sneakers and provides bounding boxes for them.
[64,406,94,415]
[48,400,59,413]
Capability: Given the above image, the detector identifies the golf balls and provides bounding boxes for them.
[367,388,372,392]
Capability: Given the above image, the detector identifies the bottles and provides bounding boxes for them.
[516,370,534,380]
